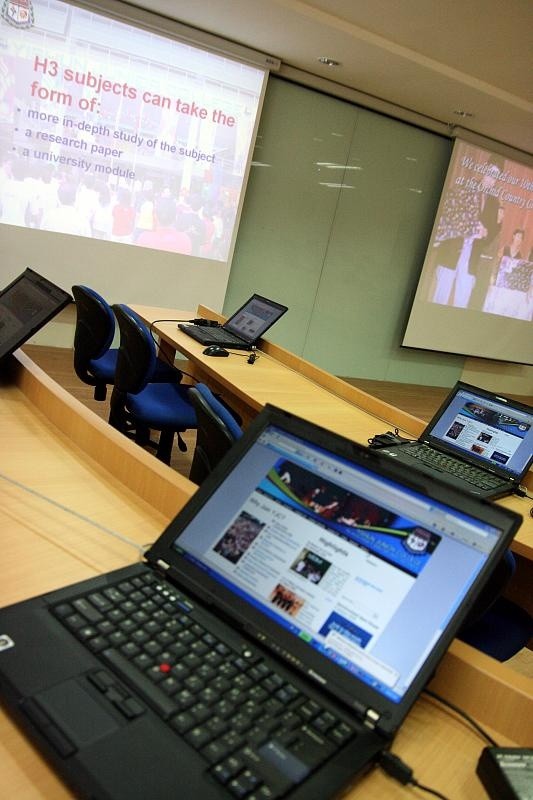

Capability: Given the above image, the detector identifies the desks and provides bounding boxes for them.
[0,347,533,800]
[126,303,533,559]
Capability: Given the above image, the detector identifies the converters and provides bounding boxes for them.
[475,746,533,800]
[193,318,219,328]
[373,433,410,448]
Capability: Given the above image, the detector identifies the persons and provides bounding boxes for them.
[214,518,257,561]
[0,151,235,259]
[308,484,341,514]
[272,588,296,613]
[431,153,524,309]
[297,559,322,582]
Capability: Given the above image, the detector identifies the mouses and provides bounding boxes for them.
[203,345,230,357]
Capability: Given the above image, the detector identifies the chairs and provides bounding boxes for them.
[72,285,183,449]
[111,303,242,467]
[454,550,524,663]
[188,383,243,487]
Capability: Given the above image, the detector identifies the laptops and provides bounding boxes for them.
[0,402,523,800]
[178,293,289,350]
[374,380,533,504]
[0,266,73,366]
